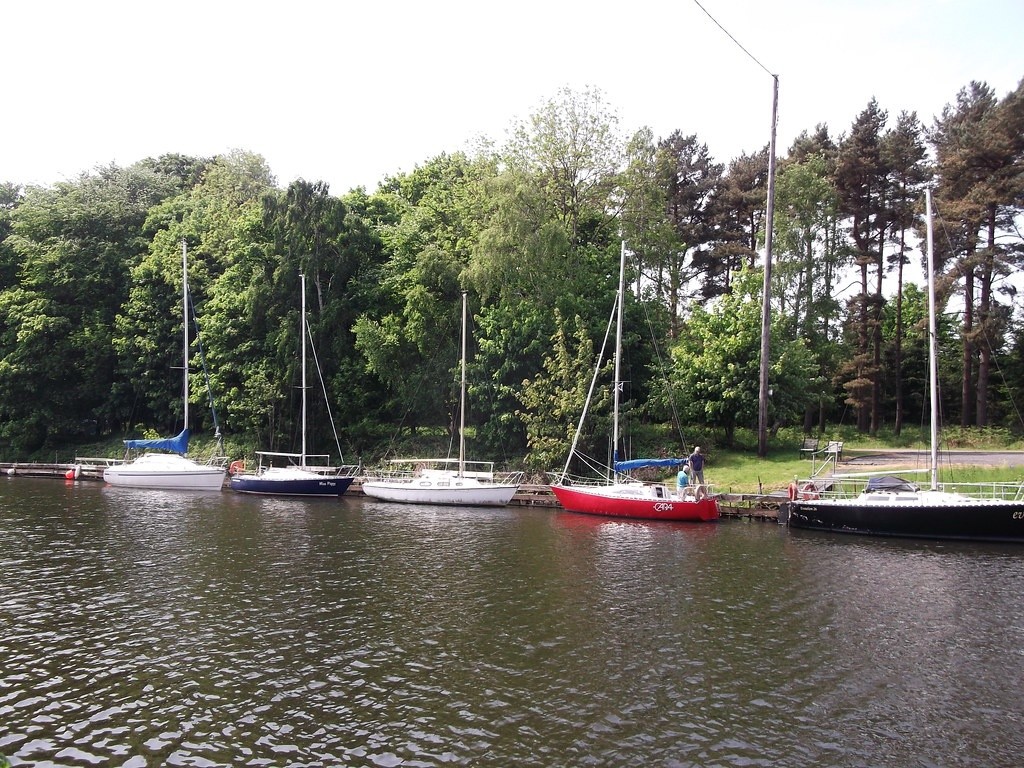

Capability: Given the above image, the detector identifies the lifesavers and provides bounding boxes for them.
[230,461,242,474]
[803,484,819,500]
[788,483,797,500]
[695,485,707,501]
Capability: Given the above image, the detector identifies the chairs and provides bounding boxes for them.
[823,441,843,462]
[800,439,818,460]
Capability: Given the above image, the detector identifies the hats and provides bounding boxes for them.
[696,447,700,452]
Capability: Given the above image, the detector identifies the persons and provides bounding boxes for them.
[676,465,690,501]
[689,447,709,496]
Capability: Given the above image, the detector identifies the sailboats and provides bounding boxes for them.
[230,274,359,497]
[546,240,721,521]
[788,189,1023,542]
[104,238,230,491]
[361,293,524,505]
